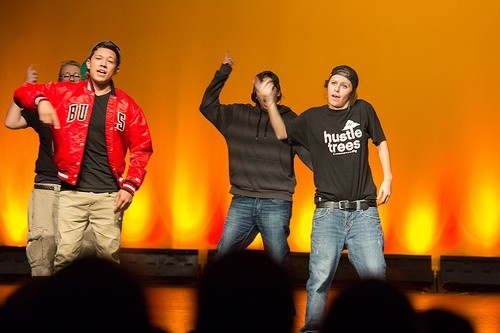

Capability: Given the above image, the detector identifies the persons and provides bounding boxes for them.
[5,60,83,277]
[199,51,315,267]
[13,39,153,277]
[0,251,473,333]
[255,64,394,333]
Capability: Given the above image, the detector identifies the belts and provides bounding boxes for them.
[34,183,54,190]
[315,200,376,210]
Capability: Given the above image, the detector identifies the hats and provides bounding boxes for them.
[330,65,359,91]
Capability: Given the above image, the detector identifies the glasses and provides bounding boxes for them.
[60,72,83,80]
